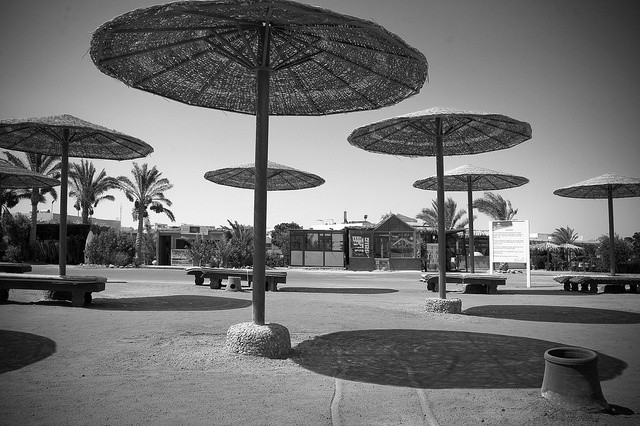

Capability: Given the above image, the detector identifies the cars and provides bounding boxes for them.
[578,258,598,270]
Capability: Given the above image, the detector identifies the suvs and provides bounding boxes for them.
[571,256,587,268]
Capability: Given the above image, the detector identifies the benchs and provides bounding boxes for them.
[1,272,108,304]
[553,275,573,290]
[185,266,287,292]
[0,261,33,274]
[422,271,508,294]
[569,274,640,294]
